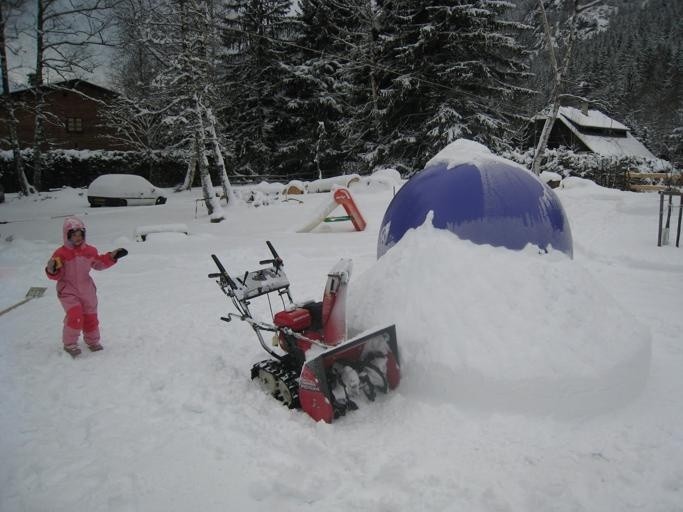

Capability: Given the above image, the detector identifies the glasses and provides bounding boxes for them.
[69,228,84,232]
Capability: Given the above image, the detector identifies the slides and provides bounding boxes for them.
[298,193,338,233]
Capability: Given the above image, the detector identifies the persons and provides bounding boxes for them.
[43,213,128,357]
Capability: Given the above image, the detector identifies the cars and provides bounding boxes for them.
[86,173,169,206]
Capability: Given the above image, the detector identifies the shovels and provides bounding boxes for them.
[0,287,48,316]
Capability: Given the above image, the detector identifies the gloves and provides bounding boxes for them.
[46,259,59,276]
[110,248,128,262]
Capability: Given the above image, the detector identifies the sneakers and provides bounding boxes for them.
[63,343,81,356]
[89,344,103,352]
